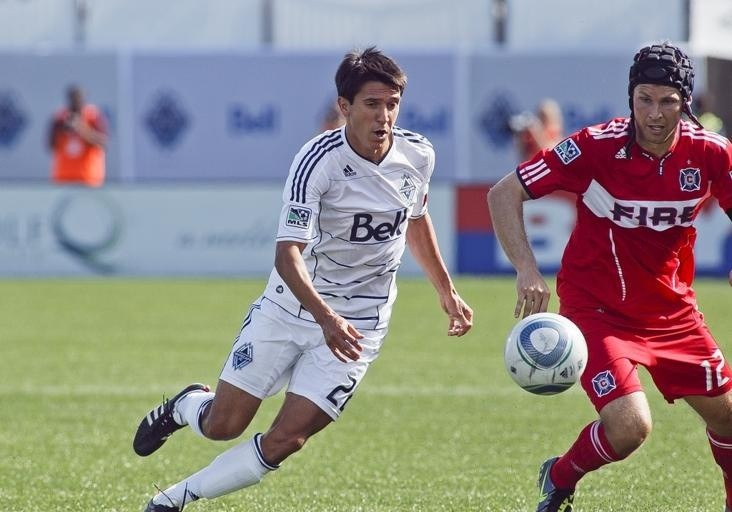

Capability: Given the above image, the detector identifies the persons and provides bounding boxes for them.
[49,82,108,189]
[512,100,565,160]
[693,95,724,133]
[486,45,732,512]
[132,47,474,512]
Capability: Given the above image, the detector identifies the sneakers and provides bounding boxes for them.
[145,499,182,512]
[134,385,209,456]
[537,458,575,512]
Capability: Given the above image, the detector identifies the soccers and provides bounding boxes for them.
[505,311,590,397]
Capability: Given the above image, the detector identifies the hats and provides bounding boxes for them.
[629,45,694,108]
